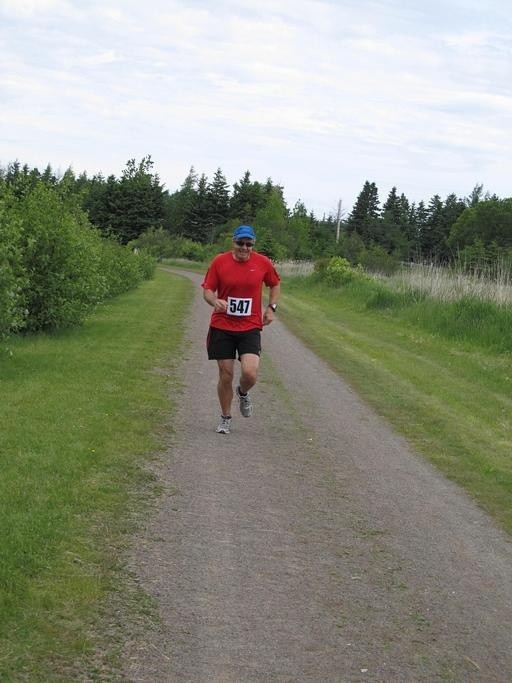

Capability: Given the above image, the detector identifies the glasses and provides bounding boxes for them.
[236,240,254,246]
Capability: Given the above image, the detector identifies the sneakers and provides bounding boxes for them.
[236,386,252,417]
[216,415,232,433]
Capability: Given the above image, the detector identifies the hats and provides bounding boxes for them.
[233,225,256,240]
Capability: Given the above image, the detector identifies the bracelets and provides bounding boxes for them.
[267,303,278,311]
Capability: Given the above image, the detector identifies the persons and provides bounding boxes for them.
[200,224,281,434]
